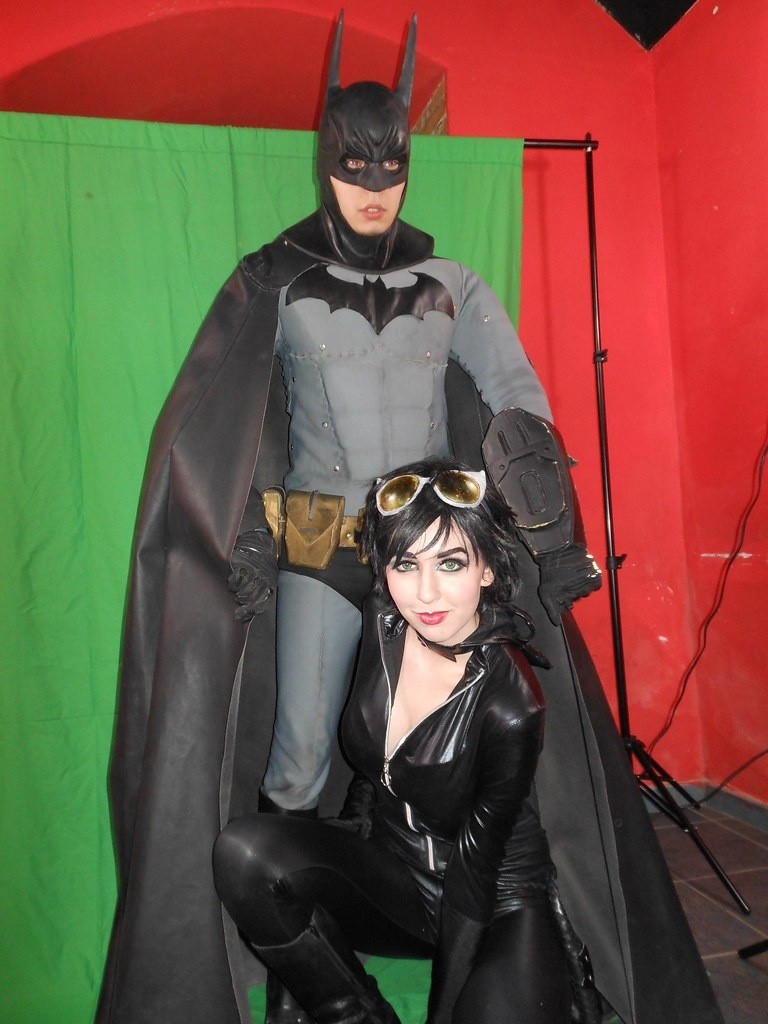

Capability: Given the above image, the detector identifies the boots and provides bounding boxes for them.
[252,898,404,1024]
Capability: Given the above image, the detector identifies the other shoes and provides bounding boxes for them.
[264,967,309,1024]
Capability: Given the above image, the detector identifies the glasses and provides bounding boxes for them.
[375,470,486,517]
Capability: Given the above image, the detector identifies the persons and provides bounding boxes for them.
[149,82,605,1024]
[211,453,590,1024]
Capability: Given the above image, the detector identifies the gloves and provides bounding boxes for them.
[227,531,281,622]
[535,543,603,627]
